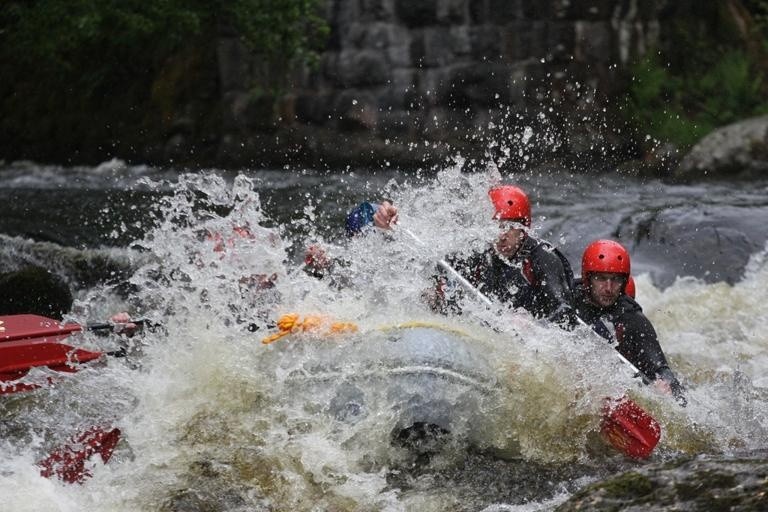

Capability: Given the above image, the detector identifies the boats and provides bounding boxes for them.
[313,323,526,462]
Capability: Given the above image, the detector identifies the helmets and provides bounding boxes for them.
[489,186,531,229]
[582,240,635,300]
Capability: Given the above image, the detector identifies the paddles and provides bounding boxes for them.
[0,343,126,397]
[0,315,161,347]
[35,422,120,485]
[393,219,660,460]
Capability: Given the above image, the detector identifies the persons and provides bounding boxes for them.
[111,220,337,339]
[341,182,578,339]
[562,240,691,411]
